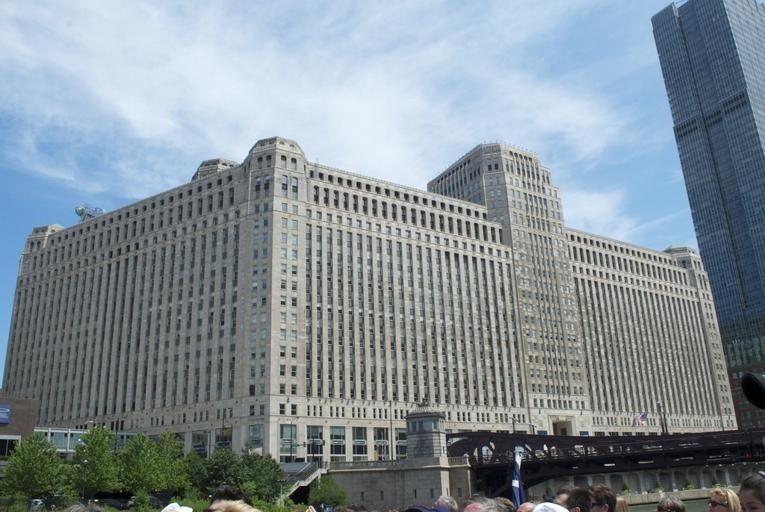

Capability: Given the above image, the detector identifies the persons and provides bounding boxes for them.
[658,496,685,512]
[210,485,259,511]
[406,482,628,512]
[707,472,765,511]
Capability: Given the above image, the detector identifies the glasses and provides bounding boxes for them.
[590,502,601,508]
[707,499,728,508]
